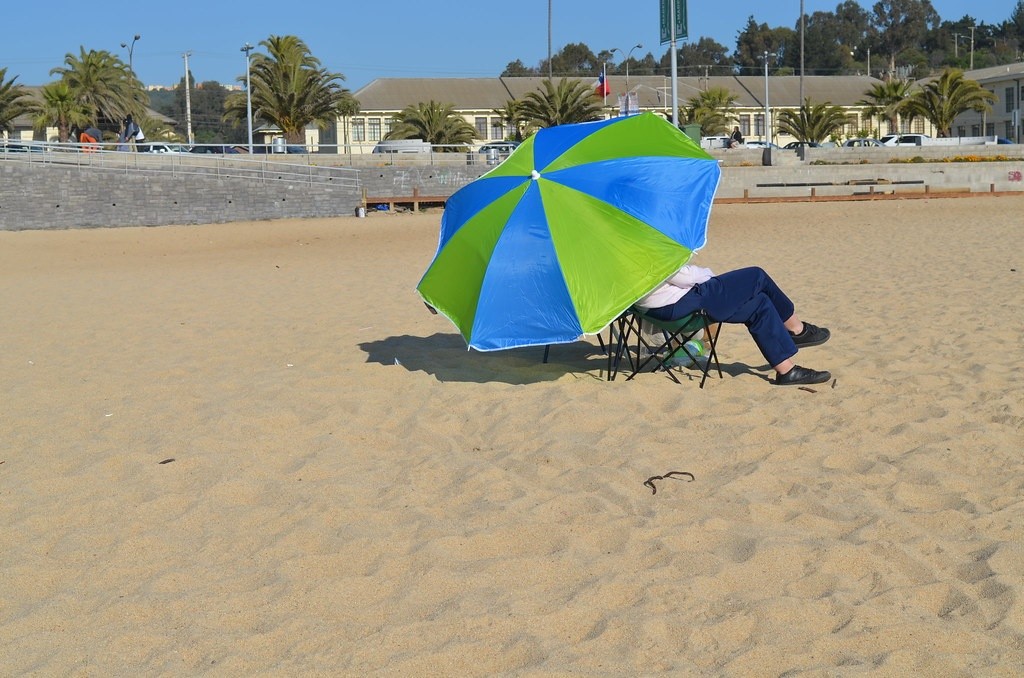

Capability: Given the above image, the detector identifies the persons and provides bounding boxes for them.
[122,113,146,153]
[727,126,743,149]
[631,261,831,386]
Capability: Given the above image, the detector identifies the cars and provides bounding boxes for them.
[470,141,520,152]
[134,142,308,155]
[701,134,930,149]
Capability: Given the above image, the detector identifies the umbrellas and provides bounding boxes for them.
[414,113,724,353]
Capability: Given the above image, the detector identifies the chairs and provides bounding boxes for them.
[543,305,723,388]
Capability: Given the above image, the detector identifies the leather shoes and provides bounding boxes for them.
[776,365,831,385]
[788,321,830,347]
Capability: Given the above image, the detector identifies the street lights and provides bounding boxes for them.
[610,43,643,115]
[755,50,776,148]
[178,51,192,143]
[120,35,141,113]
[240,42,254,153]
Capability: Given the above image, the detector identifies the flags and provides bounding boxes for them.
[592,67,611,99]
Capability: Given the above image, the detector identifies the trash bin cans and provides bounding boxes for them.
[271,135,286,152]
[681,124,702,146]
[487,148,500,165]
